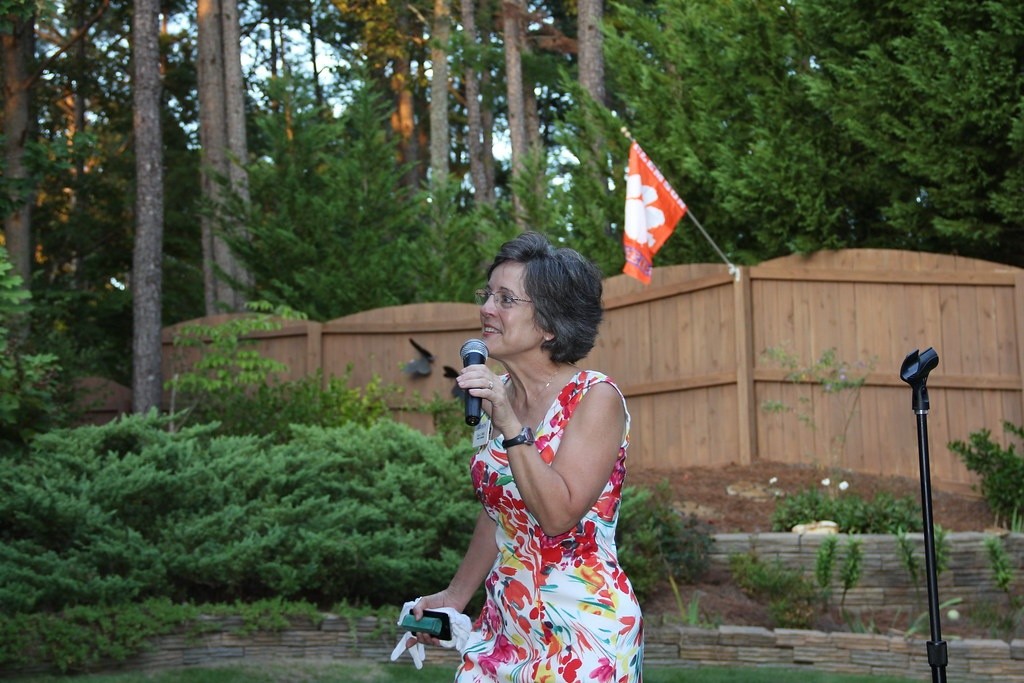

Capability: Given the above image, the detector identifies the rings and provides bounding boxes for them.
[488,380,495,390]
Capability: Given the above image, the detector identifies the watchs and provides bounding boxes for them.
[502,426,535,448]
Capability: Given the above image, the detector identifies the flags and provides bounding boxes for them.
[620,138,685,286]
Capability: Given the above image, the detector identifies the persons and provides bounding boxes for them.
[401,231,646,683]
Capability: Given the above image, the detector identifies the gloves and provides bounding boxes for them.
[390,597,473,670]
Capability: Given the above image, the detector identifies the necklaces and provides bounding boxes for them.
[513,360,562,412]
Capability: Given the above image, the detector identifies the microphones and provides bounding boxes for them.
[460,338,490,427]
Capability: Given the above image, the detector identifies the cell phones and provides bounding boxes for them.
[409,608,452,641]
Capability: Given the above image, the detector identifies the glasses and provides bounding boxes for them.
[473,287,534,311]
